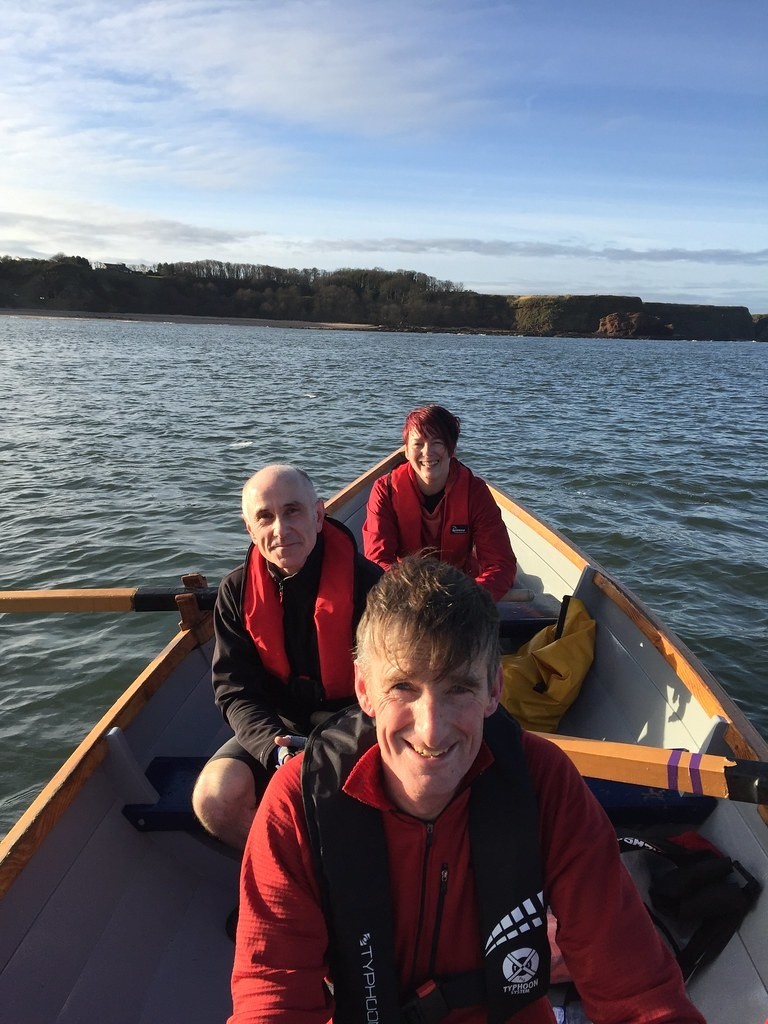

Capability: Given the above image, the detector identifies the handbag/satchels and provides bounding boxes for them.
[614,832,762,981]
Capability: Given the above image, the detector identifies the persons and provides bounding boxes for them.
[226,555,709,1024]
[190,464,389,849]
[362,406,517,620]
